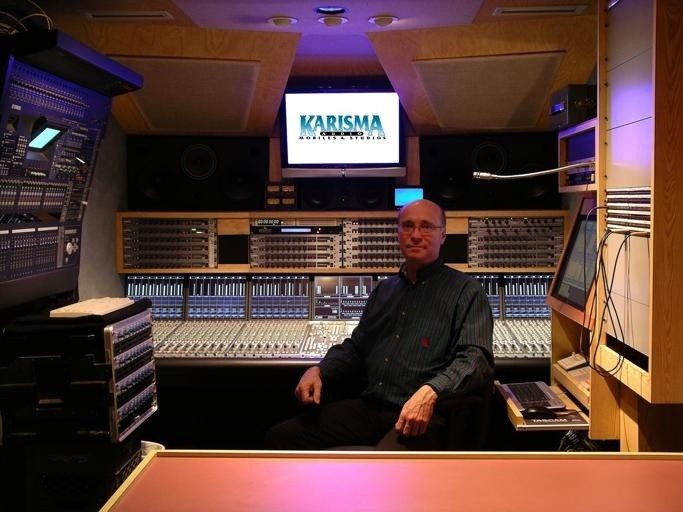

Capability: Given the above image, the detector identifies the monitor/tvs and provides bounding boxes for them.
[549,212,599,312]
[282,88,407,179]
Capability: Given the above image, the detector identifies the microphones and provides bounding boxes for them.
[473,162,596,180]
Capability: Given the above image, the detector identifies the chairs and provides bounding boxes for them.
[430,388,493,451]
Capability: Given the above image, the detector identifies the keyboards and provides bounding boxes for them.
[502,381,566,412]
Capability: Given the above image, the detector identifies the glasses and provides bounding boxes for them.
[398,222,444,233]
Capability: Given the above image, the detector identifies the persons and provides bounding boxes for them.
[261,199,494,452]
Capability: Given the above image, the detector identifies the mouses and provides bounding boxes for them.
[522,406,558,419]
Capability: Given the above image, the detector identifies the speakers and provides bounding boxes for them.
[418,130,563,211]
[295,177,396,211]
[126,132,270,212]
